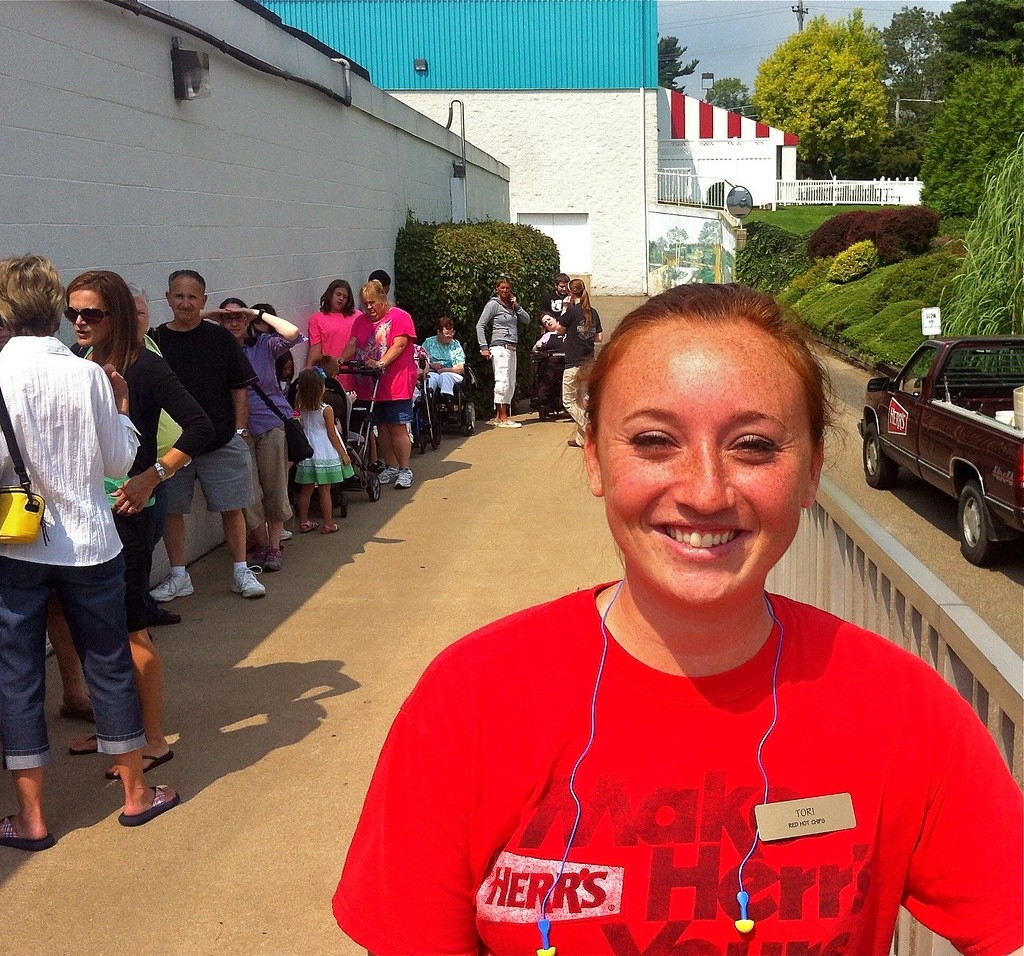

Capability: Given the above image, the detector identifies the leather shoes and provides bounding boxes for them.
[149,608,182,626]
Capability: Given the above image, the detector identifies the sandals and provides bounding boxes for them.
[298,519,320,533]
[321,522,340,534]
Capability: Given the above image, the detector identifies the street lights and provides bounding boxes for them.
[895,93,945,125]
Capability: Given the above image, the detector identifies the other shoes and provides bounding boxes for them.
[568,440,581,447]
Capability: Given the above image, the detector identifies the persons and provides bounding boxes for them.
[201,297,308,572]
[0,253,180,851]
[421,316,465,397]
[556,279,603,448]
[46,269,217,780]
[533,272,570,403]
[294,269,417,533]
[476,276,530,428]
[148,270,266,600]
[330,284,1024,956]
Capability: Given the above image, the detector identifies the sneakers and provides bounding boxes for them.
[377,465,401,484]
[263,550,285,571]
[366,460,385,474]
[393,467,415,489]
[498,419,523,428]
[231,565,266,598]
[247,550,268,571]
[493,418,514,426]
[148,570,195,602]
[265,522,294,541]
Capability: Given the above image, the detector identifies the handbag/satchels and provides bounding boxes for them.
[0,485,49,545]
[284,417,315,462]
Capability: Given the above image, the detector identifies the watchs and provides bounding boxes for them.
[153,462,167,481]
[235,429,248,437]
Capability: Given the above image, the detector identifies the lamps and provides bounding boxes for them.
[171,49,211,100]
[414,59,427,71]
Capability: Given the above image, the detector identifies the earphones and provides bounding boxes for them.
[735,892,754,933]
[537,920,555,956]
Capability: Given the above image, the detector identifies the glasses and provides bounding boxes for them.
[63,306,113,326]
[222,315,246,323]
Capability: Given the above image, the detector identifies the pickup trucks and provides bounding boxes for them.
[857,331,1024,567]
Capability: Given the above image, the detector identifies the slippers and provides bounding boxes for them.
[104,746,174,780]
[57,695,98,724]
[118,785,181,827]
[0,815,56,852]
[68,733,100,755]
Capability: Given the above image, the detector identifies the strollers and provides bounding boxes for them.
[288,359,385,519]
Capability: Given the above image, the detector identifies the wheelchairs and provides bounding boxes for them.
[426,363,476,437]
[527,350,576,422]
[411,358,442,455]
